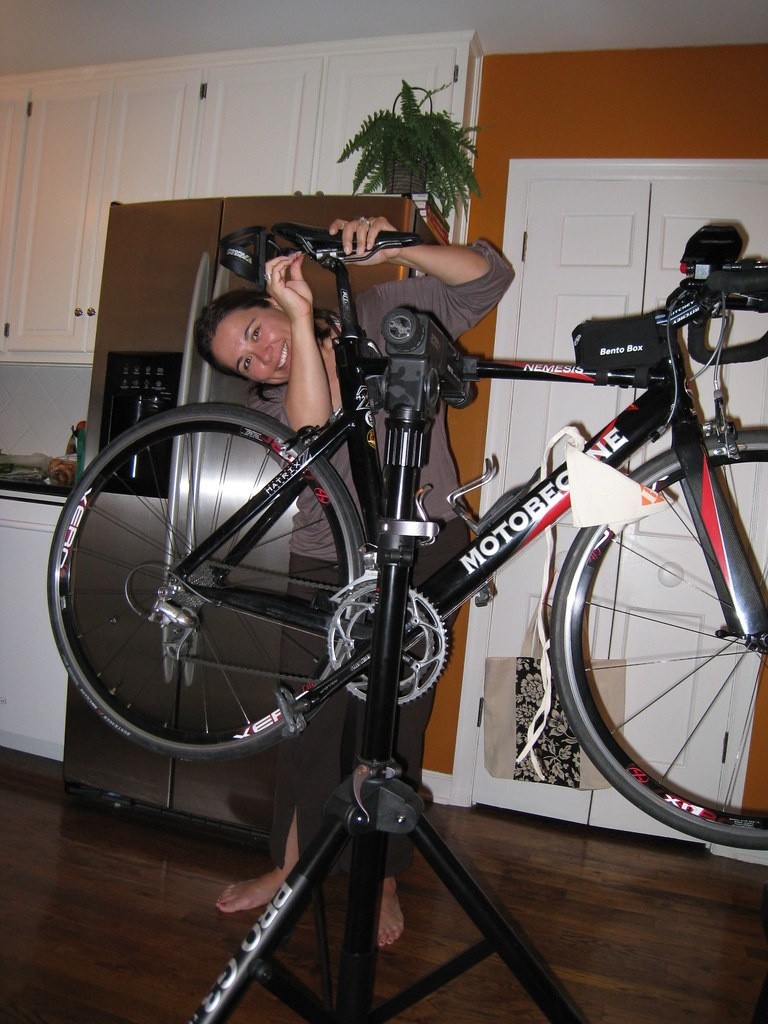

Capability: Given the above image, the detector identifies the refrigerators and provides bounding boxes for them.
[64,192,443,854]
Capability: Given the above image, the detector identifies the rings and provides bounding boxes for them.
[359,217,370,224]
[264,274,271,280]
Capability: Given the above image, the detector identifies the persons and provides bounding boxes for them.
[193,217,516,948]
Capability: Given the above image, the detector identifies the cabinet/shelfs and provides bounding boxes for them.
[0,497,71,764]
[0,26,484,368]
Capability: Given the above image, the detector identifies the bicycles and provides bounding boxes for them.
[47,219,768,850]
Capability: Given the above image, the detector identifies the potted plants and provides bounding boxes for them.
[337,74,492,219]
